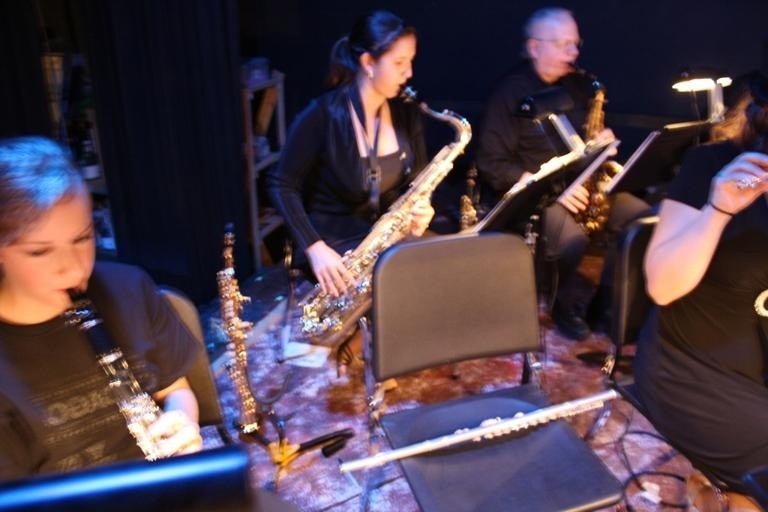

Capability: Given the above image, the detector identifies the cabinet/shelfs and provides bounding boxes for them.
[241,70,292,269]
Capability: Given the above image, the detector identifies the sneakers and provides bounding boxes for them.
[552,307,591,340]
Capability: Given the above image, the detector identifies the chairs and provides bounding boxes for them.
[585,217,747,495]
[359,232,625,512]
[158,289,239,447]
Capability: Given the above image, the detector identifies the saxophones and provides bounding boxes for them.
[303,86,471,340]
[570,63,623,231]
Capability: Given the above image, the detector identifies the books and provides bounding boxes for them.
[378,384,626,511]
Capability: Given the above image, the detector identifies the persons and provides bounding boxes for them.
[480,7,654,340]
[268,11,439,302]
[1,137,202,488]
[633,59,767,510]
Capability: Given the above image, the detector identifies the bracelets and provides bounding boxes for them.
[708,199,734,220]
